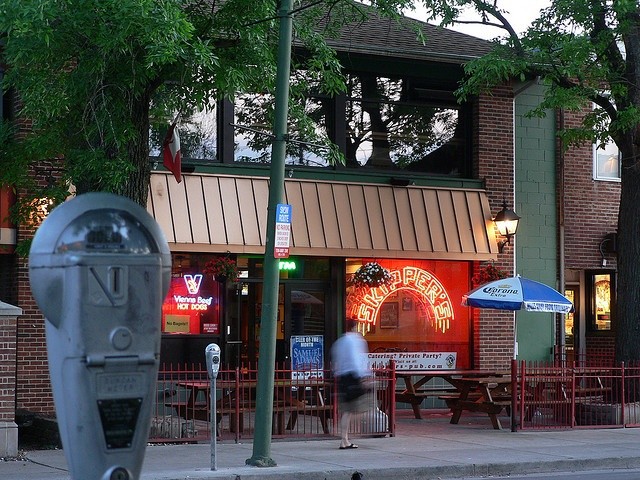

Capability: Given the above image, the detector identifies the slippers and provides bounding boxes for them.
[339,444,358,449]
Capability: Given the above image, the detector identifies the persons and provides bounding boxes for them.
[327,319,378,449]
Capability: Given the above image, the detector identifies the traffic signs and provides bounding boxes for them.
[274,204,293,258]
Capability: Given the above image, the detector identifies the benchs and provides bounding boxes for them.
[164,401,285,426]
[514,385,565,392]
[549,387,612,395]
[410,392,486,399]
[482,400,591,414]
[438,393,534,411]
[375,387,477,395]
[208,405,335,417]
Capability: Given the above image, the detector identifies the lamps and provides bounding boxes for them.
[491,197,522,252]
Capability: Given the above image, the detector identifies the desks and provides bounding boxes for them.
[176,379,327,440]
[450,376,578,430]
[516,367,609,400]
[375,369,512,418]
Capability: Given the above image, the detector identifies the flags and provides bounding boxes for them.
[163,113,182,183]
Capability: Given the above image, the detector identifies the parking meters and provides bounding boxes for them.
[28,193,172,480]
[206,343,220,380]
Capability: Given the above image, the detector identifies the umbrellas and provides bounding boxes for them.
[463,273,575,359]
